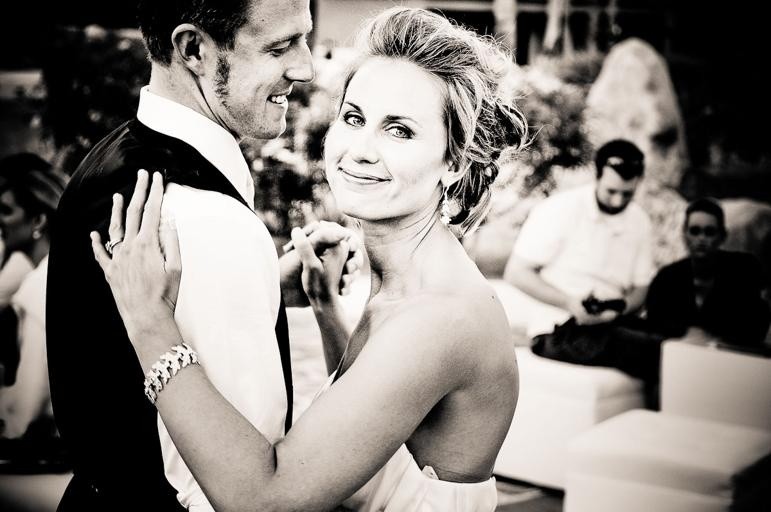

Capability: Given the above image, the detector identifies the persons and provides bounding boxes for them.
[42,1,365,511]
[645,199,771,361]
[503,139,662,411]
[1,150,77,477]
[86,5,543,511]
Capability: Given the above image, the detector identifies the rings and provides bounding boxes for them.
[107,238,126,252]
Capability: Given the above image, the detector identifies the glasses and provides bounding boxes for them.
[687,224,722,237]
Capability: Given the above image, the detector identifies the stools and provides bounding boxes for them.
[564,408,771,511]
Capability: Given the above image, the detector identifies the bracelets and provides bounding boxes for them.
[144,342,202,405]
